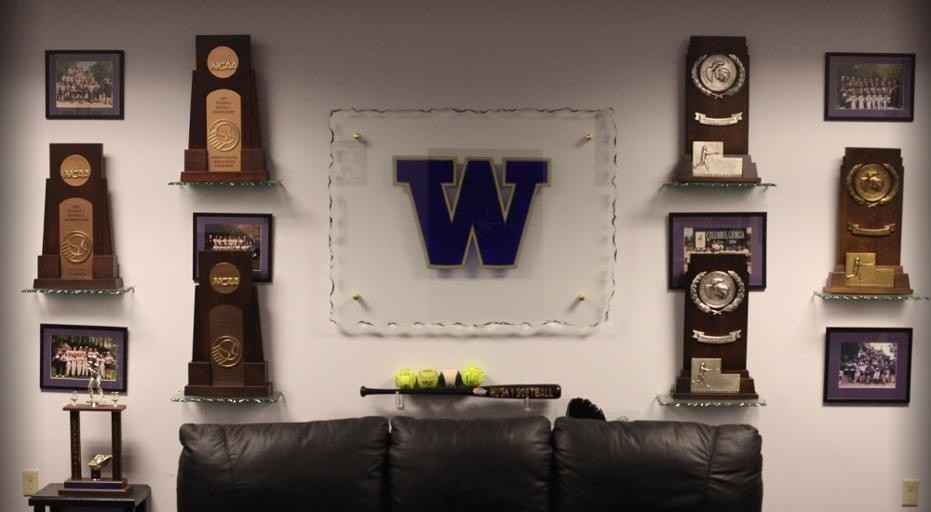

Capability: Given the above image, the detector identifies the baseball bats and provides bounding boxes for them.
[360,384,561,399]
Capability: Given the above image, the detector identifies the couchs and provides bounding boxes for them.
[176,414,764,512]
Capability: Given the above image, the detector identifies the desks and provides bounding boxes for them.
[27,482,153,511]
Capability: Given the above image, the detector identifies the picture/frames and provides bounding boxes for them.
[669,212,768,292]
[39,324,129,391]
[44,49,125,119]
[824,52,916,121]
[823,326,913,404]
[192,212,273,282]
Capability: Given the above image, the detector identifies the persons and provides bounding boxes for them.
[839,348,894,385]
[841,75,899,109]
[86,359,104,402]
[56,66,113,105]
[684,240,751,263]
[54,342,114,380]
[207,234,256,258]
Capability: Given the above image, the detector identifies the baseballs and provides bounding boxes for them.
[418,369,439,388]
[440,369,461,388]
[464,366,484,387]
[395,368,416,389]
